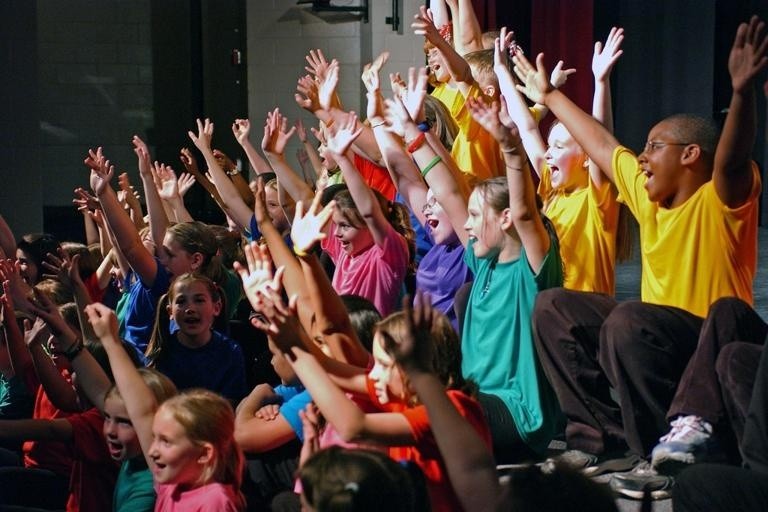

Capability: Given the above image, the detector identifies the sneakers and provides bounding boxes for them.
[541,415,717,501]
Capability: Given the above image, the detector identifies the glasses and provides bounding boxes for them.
[647,139,707,153]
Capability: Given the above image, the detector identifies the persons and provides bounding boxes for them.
[0,1,764,511]
